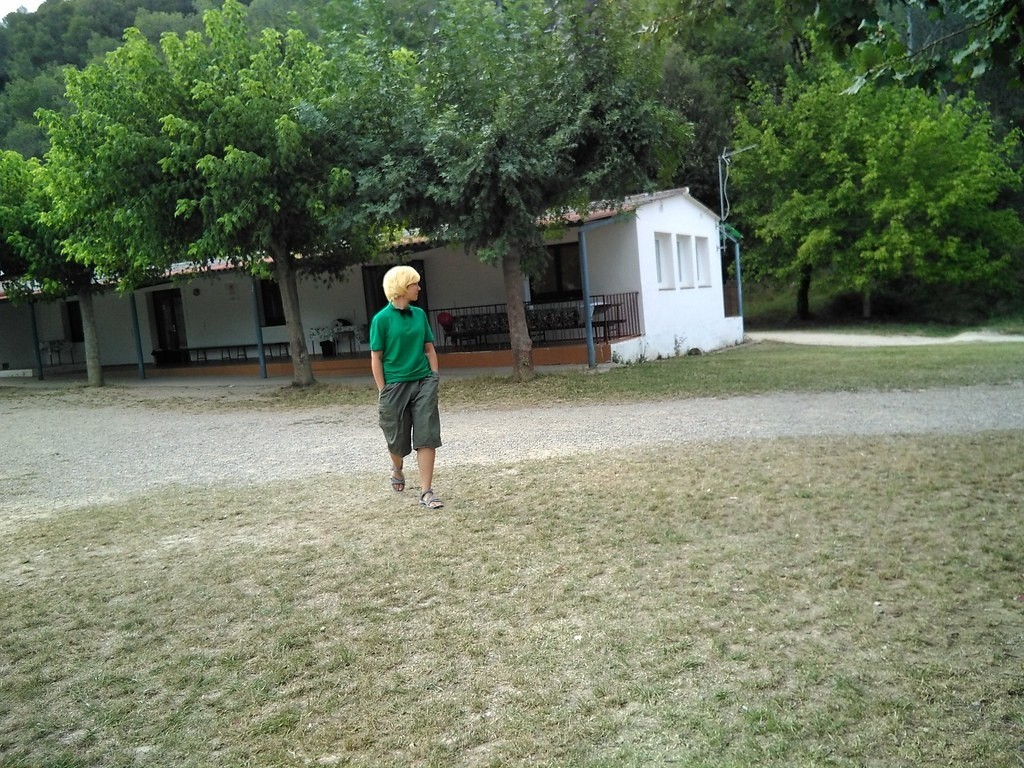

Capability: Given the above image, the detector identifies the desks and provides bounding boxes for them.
[310,323,368,357]
[452,301,626,352]
[38,340,75,366]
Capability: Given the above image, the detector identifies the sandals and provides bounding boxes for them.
[419,489,444,509]
[390,459,405,491]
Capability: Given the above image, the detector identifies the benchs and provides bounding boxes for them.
[443,319,627,352]
[151,342,290,365]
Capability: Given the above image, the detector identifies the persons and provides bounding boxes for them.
[369,265,444,509]
